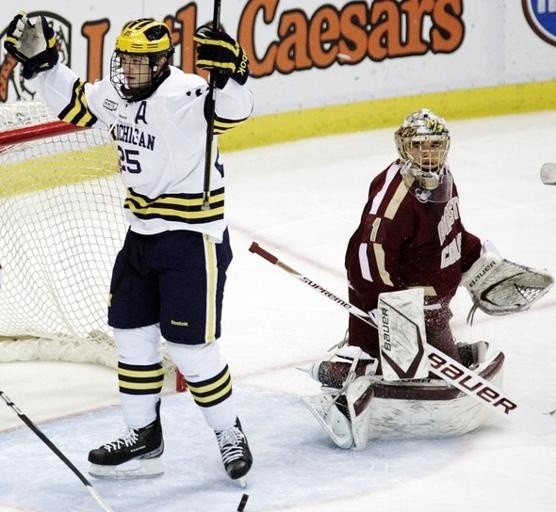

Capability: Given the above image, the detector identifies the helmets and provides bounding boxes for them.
[110,17,175,98]
[394,108,452,187]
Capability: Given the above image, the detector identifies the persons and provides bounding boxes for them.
[0,10,259,483]
[297,105,554,454]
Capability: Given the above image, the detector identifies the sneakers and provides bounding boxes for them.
[322,393,354,446]
[87,396,165,466]
[309,359,345,389]
[213,415,254,481]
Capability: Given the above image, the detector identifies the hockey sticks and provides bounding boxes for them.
[249,244,556,444]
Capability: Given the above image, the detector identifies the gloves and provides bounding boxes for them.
[3,8,60,78]
[191,26,250,86]
